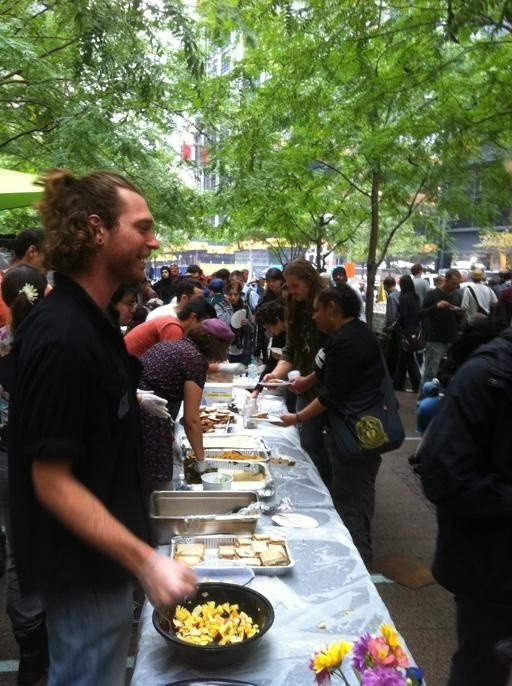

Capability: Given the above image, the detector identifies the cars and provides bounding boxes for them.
[328,260,503,306]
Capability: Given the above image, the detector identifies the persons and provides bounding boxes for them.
[383,263,511,686]
[7,161,203,684]
[116,265,260,459]
[0,228,55,353]
[251,258,395,571]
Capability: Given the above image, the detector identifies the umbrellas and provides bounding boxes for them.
[0,167,54,212]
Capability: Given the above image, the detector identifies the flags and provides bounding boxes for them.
[183,142,191,161]
[202,143,208,162]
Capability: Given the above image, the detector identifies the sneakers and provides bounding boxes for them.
[406,388,419,393]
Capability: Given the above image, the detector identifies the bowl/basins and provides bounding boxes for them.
[151,581,274,668]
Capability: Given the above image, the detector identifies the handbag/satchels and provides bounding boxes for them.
[330,380,405,466]
[400,327,426,351]
[477,305,492,316]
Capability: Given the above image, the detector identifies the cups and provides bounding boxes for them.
[288,369,301,380]
[201,470,232,490]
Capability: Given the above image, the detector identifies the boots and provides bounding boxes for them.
[15,623,49,685]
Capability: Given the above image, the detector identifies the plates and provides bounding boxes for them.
[272,512,320,531]
[248,412,284,424]
[259,379,290,387]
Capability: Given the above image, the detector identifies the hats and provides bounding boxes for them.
[470,269,484,280]
[208,278,225,289]
[201,318,235,341]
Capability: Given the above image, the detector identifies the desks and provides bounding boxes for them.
[172,388,335,507]
[128,506,426,686]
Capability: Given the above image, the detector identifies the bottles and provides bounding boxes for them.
[247,363,267,382]
[242,396,258,429]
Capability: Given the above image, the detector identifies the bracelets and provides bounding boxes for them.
[296,412,301,422]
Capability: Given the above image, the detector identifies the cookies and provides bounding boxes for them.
[172,534,289,568]
